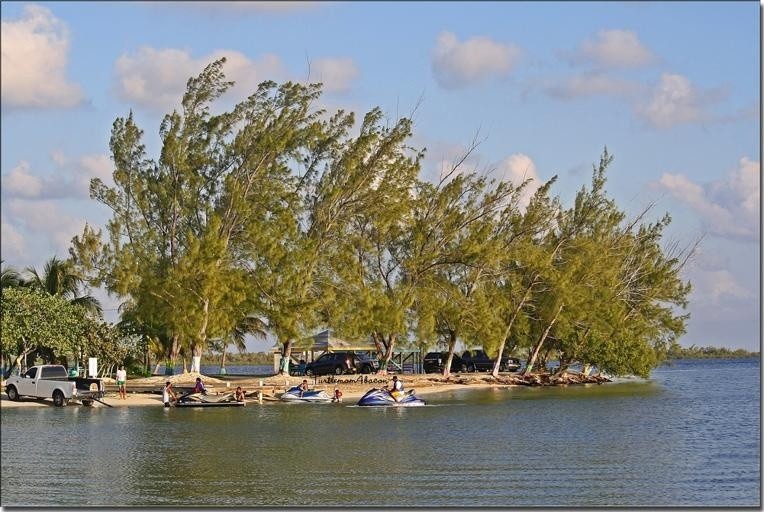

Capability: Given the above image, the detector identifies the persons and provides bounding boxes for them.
[299,380,308,397]
[234,387,245,401]
[178,378,208,403]
[299,360,305,375]
[390,376,403,392]
[289,360,294,375]
[163,382,176,407]
[332,390,343,403]
[68,368,77,381]
[115,365,127,400]
[344,351,383,374]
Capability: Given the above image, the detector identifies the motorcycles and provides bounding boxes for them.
[357,386,425,406]
[280,386,333,403]
[175,390,246,406]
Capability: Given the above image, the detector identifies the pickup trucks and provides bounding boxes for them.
[4,365,105,406]
[463,350,505,371]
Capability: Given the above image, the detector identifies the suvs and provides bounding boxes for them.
[423,352,467,373]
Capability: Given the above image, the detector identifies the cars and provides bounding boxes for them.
[305,352,379,375]
[495,357,520,371]
